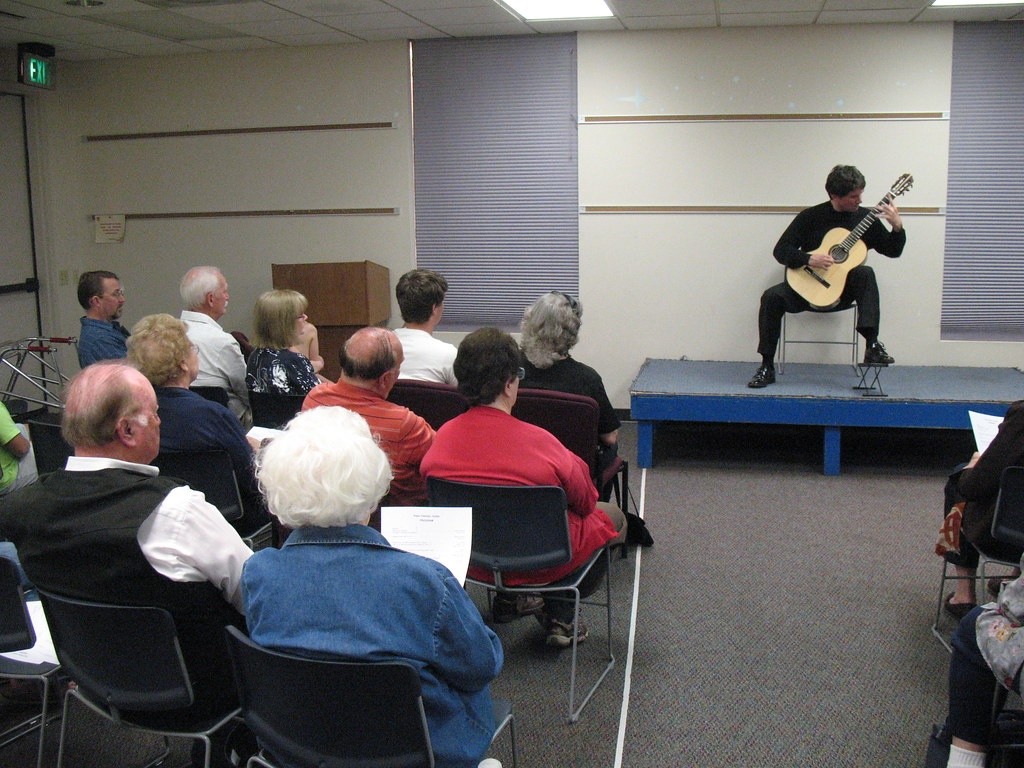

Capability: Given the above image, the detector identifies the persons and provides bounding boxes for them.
[516,290,622,503]
[300,326,437,531]
[391,268,458,388]
[237,405,504,768]
[943,399,1024,626]
[0,361,260,768]
[126,313,280,548]
[178,264,336,436]
[419,328,627,647]
[748,164,907,388]
[77,269,130,370]
[946,549,1024,768]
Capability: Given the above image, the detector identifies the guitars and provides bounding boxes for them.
[784,172,915,312]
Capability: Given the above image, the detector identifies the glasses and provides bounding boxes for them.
[191,344,199,354]
[551,290,577,309]
[515,366,525,381]
[90,288,124,304]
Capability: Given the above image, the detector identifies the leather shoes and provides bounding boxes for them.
[748,366,775,387]
[863,341,895,363]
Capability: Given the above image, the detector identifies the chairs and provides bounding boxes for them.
[0,379,628,768]
[931,466,1024,768]
[778,304,861,377]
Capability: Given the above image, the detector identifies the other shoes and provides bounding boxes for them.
[943,591,978,621]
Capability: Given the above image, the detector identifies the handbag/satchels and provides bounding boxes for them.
[626,512,654,546]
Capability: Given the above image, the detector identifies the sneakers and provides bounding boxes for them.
[547,621,588,643]
[492,594,544,621]
